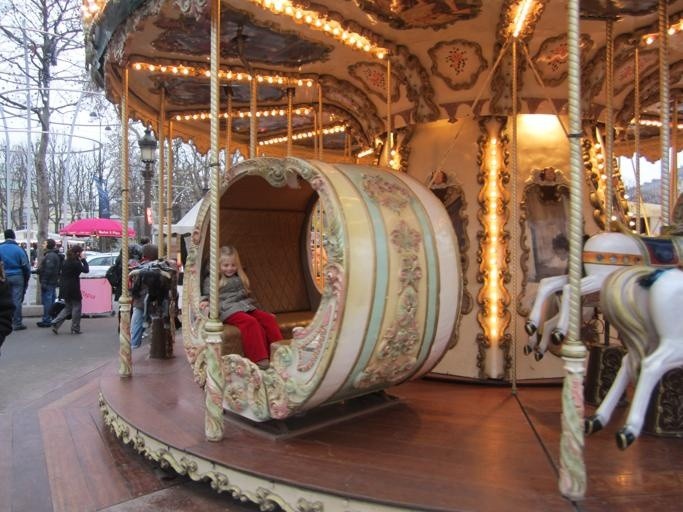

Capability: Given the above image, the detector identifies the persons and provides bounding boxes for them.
[105,235,177,350]
[47,244,89,334]
[35,238,60,327]
[17,237,63,268]
[197,244,286,368]
[0,228,30,332]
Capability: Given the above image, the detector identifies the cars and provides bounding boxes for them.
[80,251,120,279]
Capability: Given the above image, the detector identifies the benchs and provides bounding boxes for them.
[218,310,317,361]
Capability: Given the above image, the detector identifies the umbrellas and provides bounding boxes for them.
[58,217,136,251]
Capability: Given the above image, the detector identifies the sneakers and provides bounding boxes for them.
[14,321,51,330]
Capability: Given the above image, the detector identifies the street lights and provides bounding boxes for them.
[140,126,156,241]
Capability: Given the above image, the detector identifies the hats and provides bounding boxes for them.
[4,229,15,239]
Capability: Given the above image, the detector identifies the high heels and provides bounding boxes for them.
[48,322,82,335]
